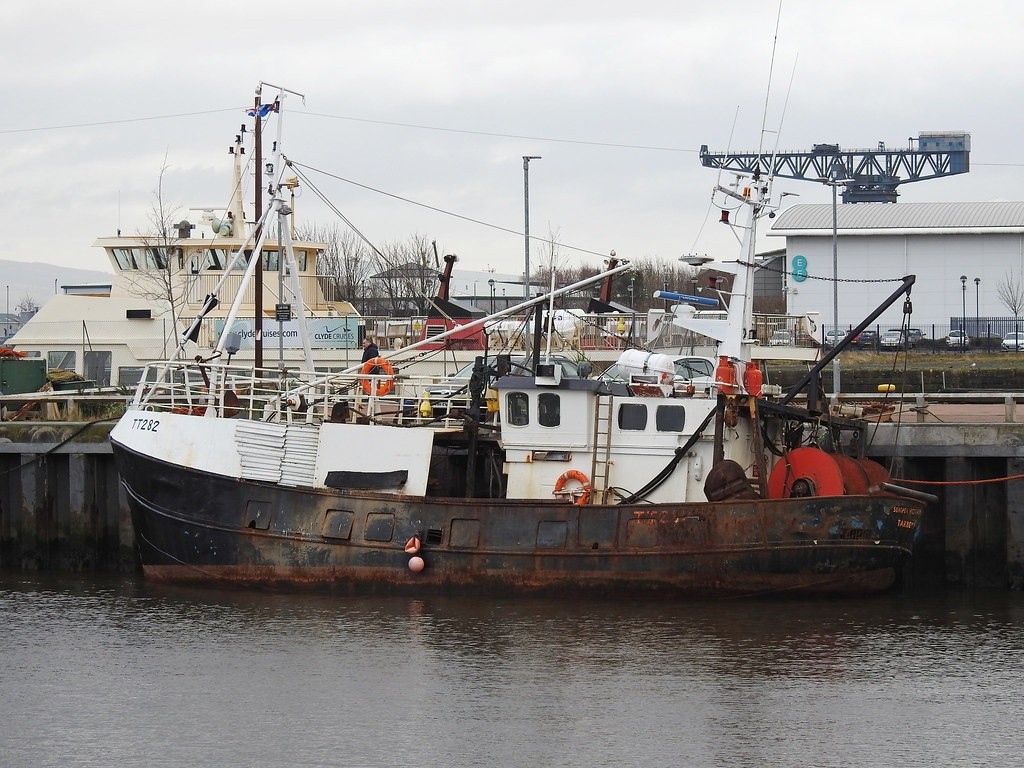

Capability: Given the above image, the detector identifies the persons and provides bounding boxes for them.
[362,337,379,375]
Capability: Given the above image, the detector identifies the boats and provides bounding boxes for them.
[1,80,942,600]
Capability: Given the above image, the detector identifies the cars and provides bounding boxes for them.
[824,329,848,348]
[857,331,876,350]
[880,330,905,351]
[423,354,582,417]
[1000,331,1024,352]
[770,332,791,345]
[847,329,863,343]
[944,329,973,349]
[907,328,926,347]
[593,356,716,394]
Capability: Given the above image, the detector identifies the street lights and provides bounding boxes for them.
[960,275,968,353]
[690,277,698,308]
[594,282,602,298]
[631,277,635,306]
[558,282,566,307]
[627,284,634,309]
[716,276,724,319]
[522,154,543,359]
[426,280,434,308]
[974,278,981,337]
[663,279,669,313]
[488,278,496,316]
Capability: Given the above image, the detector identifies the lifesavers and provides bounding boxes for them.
[360,358,394,396]
[554,470,591,505]
